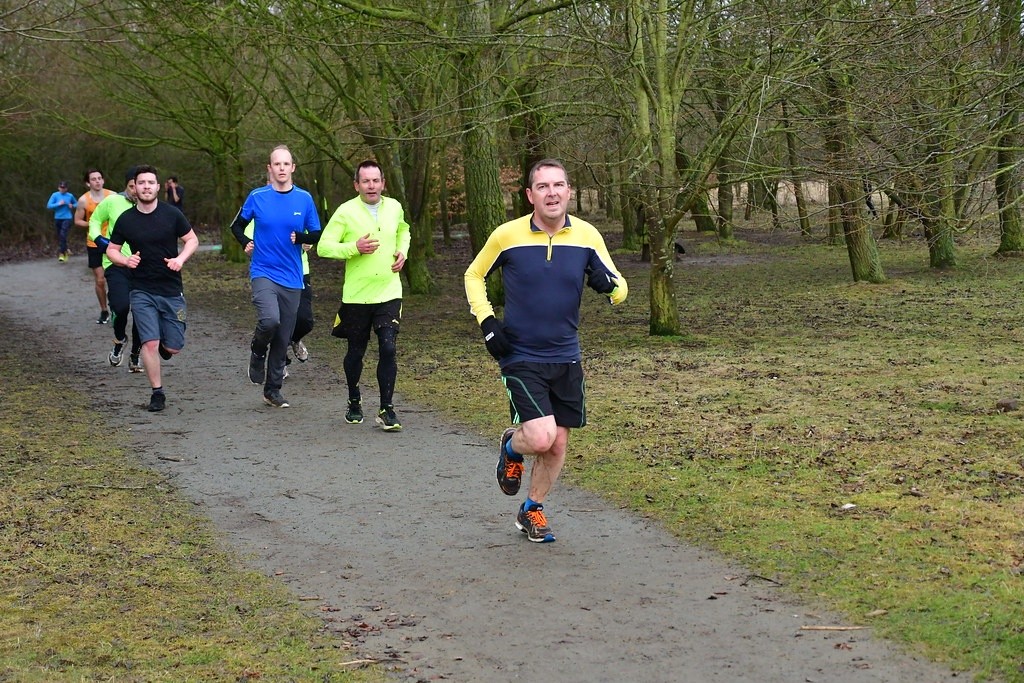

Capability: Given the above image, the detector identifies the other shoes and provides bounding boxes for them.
[58,253,69,263]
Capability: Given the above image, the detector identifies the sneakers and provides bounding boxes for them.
[263,388,290,408]
[159,341,173,361]
[497,427,525,496]
[344,394,364,424]
[108,333,129,367]
[148,391,166,412]
[282,367,288,379]
[375,404,402,432]
[127,354,144,373]
[248,351,267,385]
[515,499,556,543]
[291,340,309,362]
[96,309,110,324]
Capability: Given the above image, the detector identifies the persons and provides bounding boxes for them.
[75,165,146,371]
[108,167,199,413]
[46,181,79,262]
[316,160,412,431]
[230,144,321,408]
[464,159,628,543]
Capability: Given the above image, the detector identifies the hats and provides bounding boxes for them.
[125,166,137,183]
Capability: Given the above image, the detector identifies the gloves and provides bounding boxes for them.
[481,318,519,360]
[583,268,613,294]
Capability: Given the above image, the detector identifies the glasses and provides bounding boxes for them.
[59,186,67,189]
